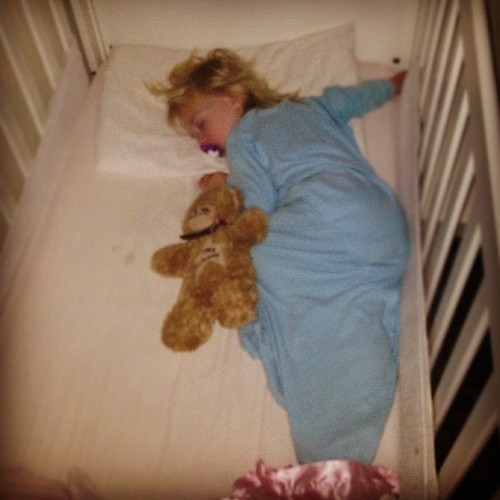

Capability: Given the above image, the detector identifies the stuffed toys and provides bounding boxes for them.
[150,186,270,354]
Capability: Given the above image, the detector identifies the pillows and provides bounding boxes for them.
[96,23,366,175]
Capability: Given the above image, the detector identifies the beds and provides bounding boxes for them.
[0,0,499,500]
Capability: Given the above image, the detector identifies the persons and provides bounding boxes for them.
[141,47,413,473]
